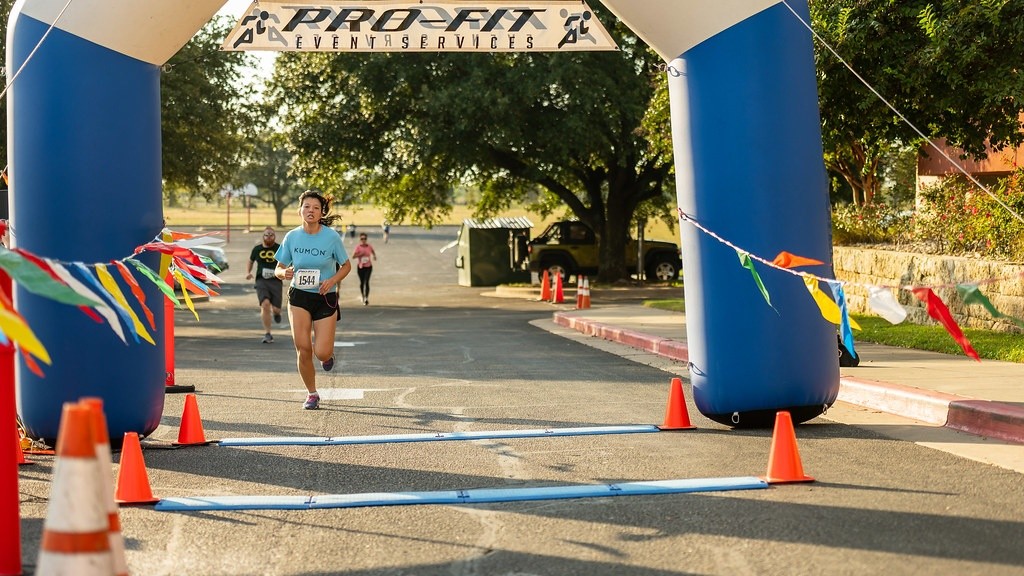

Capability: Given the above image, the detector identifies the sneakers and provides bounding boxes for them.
[262,335,274,343]
[303,394,320,409]
[274,314,281,323]
[321,353,335,372]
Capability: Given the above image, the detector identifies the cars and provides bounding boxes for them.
[170,244,229,293]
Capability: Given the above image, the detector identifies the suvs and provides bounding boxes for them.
[526,218,683,285]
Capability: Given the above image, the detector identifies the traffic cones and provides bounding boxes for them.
[580,277,591,310]
[551,272,568,304]
[536,270,553,301]
[171,394,220,447]
[572,274,584,310]
[78,395,132,576]
[655,377,699,431]
[115,432,160,505]
[31,403,116,576]
[759,410,816,486]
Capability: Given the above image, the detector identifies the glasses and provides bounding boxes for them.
[263,232,274,237]
[361,238,366,240]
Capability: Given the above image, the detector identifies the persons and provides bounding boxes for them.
[383,220,390,243]
[352,233,377,306]
[349,223,357,237]
[246,227,283,344]
[342,224,347,237]
[273,190,352,410]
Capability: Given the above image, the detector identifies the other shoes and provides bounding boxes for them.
[365,300,368,305]
